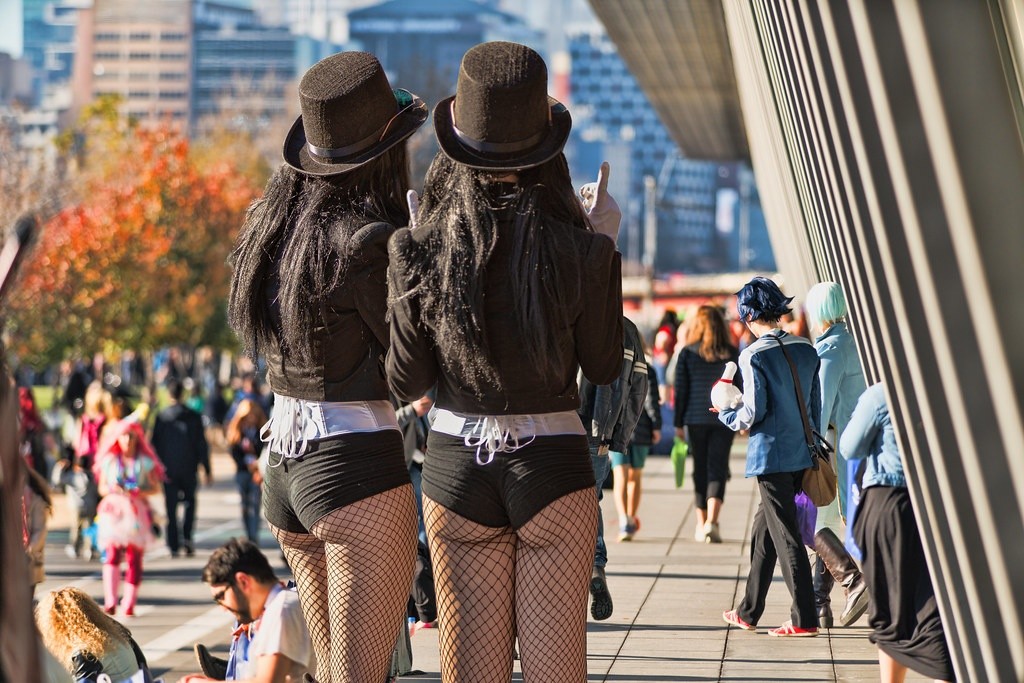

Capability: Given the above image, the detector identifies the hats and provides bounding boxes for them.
[281,51,430,178]
[434,41,572,171]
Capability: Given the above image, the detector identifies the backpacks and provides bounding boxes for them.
[76,416,102,457]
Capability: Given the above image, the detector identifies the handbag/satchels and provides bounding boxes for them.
[671,437,688,488]
[794,491,817,547]
[800,444,837,507]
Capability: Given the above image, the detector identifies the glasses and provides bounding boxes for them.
[211,579,237,604]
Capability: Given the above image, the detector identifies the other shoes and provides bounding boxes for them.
[194,644,228,681]
[619,519,638,541]
[591,567,614,621]
[704,522,722,543]
[695,525,706,544]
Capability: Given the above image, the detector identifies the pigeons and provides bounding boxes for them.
[711,361,743,414]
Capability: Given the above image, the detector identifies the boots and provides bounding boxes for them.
[813,526,869,627]
[810,551,833,628]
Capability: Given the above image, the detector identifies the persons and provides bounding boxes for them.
[35,587,152,683]
[12,365,274,615]
[229,52,418,683]
[609,333,662,543]
[706,277,823,638]
[392,394,437,635]
[805,281,870,629]
[645,299,811,359]
[839,382,956,683]
[576,318,648,621]
[176,538,318,683]
[673,304,743,543]
[385,41,625,683]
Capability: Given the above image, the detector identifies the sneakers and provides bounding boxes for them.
[768,621,819,637]
[723,608,754,630]
